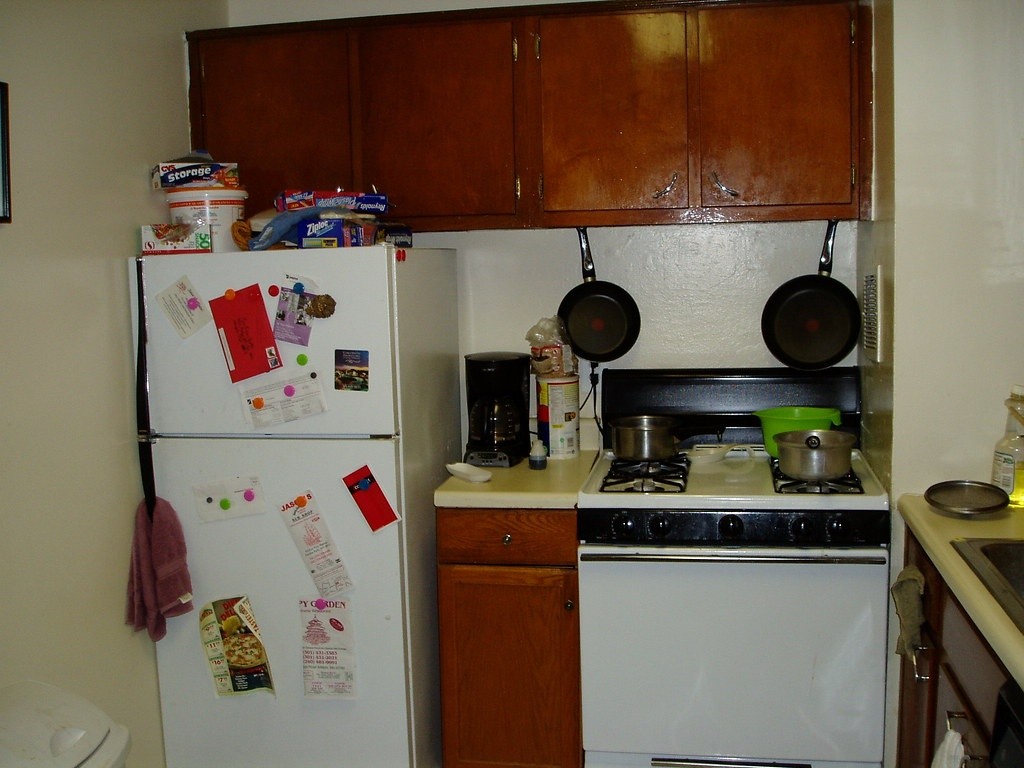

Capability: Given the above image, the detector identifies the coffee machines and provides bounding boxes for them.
[463,352,533,468]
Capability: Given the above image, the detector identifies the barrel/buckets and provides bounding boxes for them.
[167,186,251,251]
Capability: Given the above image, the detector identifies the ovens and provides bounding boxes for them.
[578,543,889,768]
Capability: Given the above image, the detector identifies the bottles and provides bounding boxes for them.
[991,386,1024,505]
[529,440,547,470]
[535,374,580,459]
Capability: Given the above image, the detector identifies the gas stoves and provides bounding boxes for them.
[574,367,890,547]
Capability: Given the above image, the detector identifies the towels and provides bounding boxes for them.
[125,493,194,642]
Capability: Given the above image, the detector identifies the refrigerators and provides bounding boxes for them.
[127,248,458,768]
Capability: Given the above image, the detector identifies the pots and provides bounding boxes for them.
[610,416,675,459]
[772,429,856,480]
[752,407,842,456]
[558,226,641,361]
[761,220,864,367]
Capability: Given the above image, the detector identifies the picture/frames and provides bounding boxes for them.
[0,82,13,224]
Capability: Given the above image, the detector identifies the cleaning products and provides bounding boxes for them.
[992,384,1024,507]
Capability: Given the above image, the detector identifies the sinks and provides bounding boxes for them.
[949,537,1024,636]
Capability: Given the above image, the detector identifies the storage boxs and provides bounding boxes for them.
[151,161,239,190]
[141,225,212,255]
[351,226,363,247]
[297,218,344,248]
[274,188,389,214]
[374,223,413,248]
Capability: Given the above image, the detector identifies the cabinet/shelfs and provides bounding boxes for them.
[434,508,583,768]
[525,0,863,229]
[188,5,525,234]
[896,523,1024,768]
